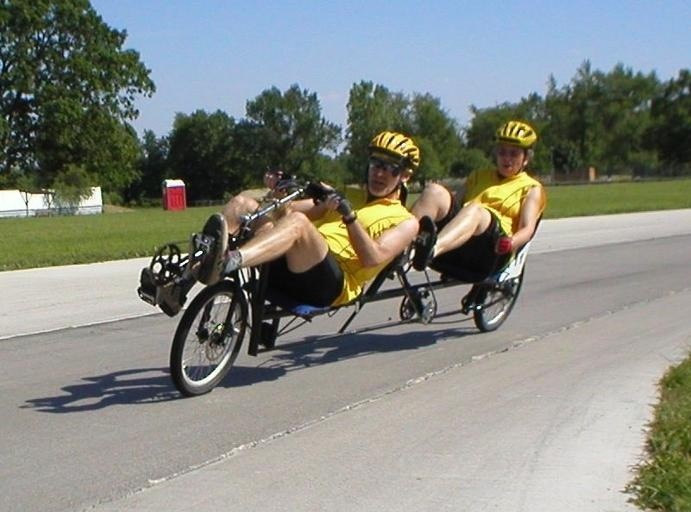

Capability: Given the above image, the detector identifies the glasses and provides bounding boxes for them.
[369,156,400,173]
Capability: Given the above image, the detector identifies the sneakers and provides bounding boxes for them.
[413,216,439,271]
[198,212,228,285]
[140,267,188,317]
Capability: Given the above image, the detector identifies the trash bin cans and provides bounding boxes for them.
[162,179,186,210]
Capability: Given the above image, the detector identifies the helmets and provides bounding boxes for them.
[495,121,536,149]
[370,130,421,174]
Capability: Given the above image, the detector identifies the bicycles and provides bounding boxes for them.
[138,169,543,396]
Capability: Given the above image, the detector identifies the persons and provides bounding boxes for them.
[140,131,422,317]
[408,121,547,284]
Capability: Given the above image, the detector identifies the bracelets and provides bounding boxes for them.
[342,211,357,225]
